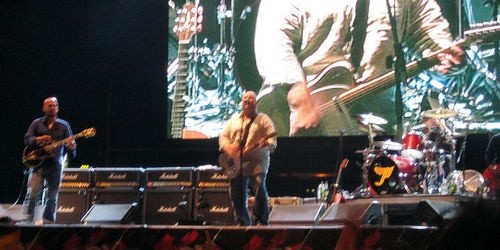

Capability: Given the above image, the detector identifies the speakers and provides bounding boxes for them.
[414,199,463,227]
[90,167,145,225]
[80,203,139,225]
[42,167,93,224]
[319,201,382,225]
[142,167,196,227]
[193,164,237,226]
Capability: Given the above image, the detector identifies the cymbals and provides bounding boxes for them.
[355,125,385,133]
[352,115,388,124]
[374,141,402,150]
[421,110,458,117]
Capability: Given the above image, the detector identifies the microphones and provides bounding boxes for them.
[244,101,247,107]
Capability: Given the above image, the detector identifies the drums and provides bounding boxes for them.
[401,132,425,158]
[365,156,416,195]
[457,171,484,194]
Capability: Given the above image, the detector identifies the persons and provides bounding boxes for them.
[416,116,452,149]
[254,0,465,136]
[0,159,500,250]
[218,91,277,227]
[20,97,77,224]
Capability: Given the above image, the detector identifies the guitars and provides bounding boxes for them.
[255,20,500,137]
[170,0,207,139]
[219,130,279,179]
[327,159,349,209]
[23,128,96,168]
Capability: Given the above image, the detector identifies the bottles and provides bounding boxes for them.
[324,181,329,197]
[448,178,457,194]
[320,181,323,198]
